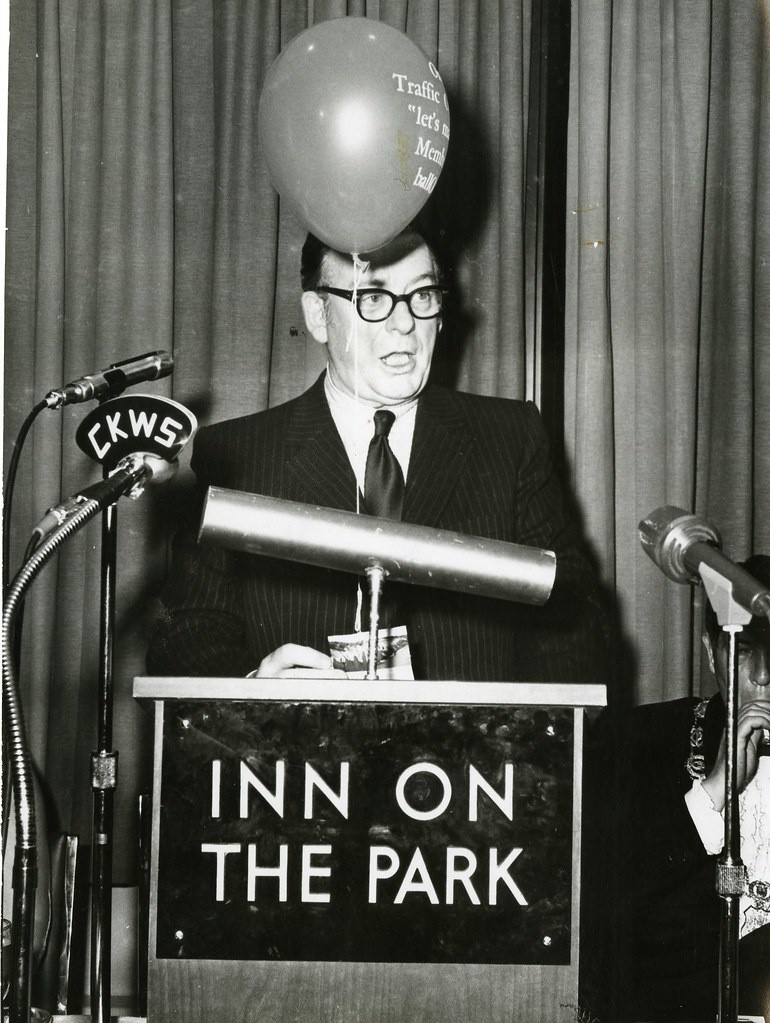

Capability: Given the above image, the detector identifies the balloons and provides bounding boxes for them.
[258,15,450,258]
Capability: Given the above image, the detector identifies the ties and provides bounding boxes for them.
[359,409,405,632]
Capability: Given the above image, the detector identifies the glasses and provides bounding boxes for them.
[313,285,449,323]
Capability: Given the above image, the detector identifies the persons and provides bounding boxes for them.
[144,232,612,684]
[584,556,770,1023]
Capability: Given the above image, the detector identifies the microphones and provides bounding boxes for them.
[639,504,770,616]
[32,453,180,544]
[44,350,175,411]
